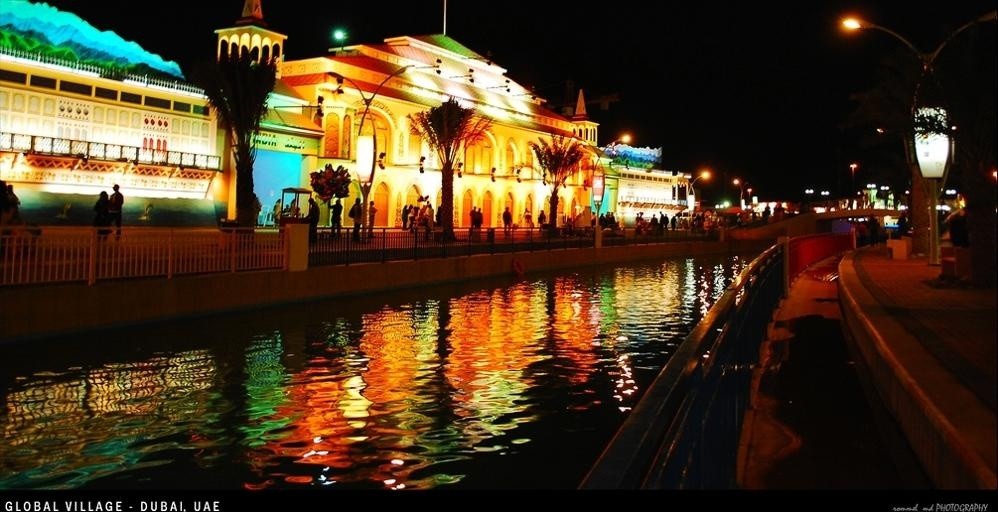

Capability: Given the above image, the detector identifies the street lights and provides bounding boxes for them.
[673,171,753,233]
[842,16,995,265]
[323,63,417,239]
[565,131,629,247]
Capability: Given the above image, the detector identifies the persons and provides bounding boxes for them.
[897,213,906,233]
[254,194,483,243]
[93,185,124,241]
[591,212,705,236]
[861,216,880,240]
[502,206,512,231]
[524,208,532,227]
[538,210,546,232]
[0,181,19,225]
